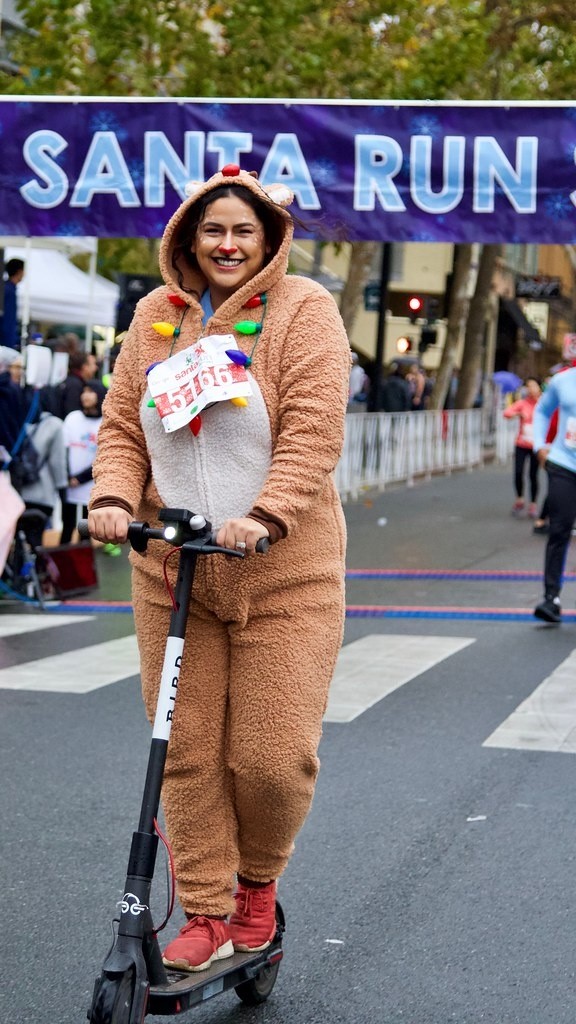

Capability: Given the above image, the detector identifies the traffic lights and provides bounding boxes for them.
[388,290,447,320]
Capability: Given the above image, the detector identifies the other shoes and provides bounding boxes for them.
[513,501,524,510]
[534,600,563,623]
[529,504,537,518]
[534,517,547,528]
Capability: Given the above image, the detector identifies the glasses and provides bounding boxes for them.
[8,364,25,370]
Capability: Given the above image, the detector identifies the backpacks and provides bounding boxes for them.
[7,413,49,489]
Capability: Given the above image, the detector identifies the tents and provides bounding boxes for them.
[3,248,122,390]
[1,236,98,353]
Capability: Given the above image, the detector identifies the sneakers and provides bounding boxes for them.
[226,881,278,952]
[162,914,234,971]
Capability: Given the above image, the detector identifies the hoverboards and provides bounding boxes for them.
[71,505,294,1024]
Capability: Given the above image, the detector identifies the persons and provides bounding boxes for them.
[0,257,104,575]
[88,164,351,971]
[348,347,436,413]
[502,365,576,625]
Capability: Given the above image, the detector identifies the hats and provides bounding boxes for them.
[83,378,107,410]
[0,346,22,374]
[31,333,43,339]
[352,352,358,361]
